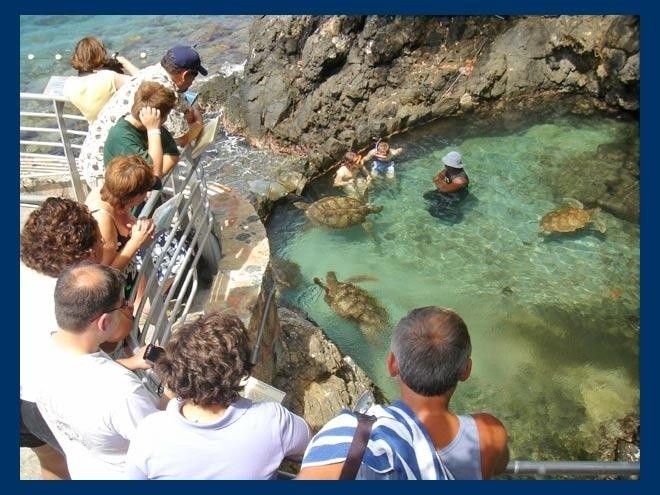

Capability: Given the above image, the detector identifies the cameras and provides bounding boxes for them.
[144,344,166,367]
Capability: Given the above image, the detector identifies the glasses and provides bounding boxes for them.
[92,298,128,323]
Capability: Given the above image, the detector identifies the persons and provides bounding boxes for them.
[32,261,178,480]
[21,196,134,482]
[103,80,196,316]
[122,312,312,481]
[424,151,468,224]
[85,154,156,359]
[333,152,371,201]
[64,36,142,127]
[290,305,510,481]
[77,45,208,192]
[363,138,403,191]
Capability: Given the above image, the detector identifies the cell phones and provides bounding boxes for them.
[183,92,198,105]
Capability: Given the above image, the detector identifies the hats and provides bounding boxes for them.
[125,171,162,198]
[441,151,464,169]
[164,45,207,76]
